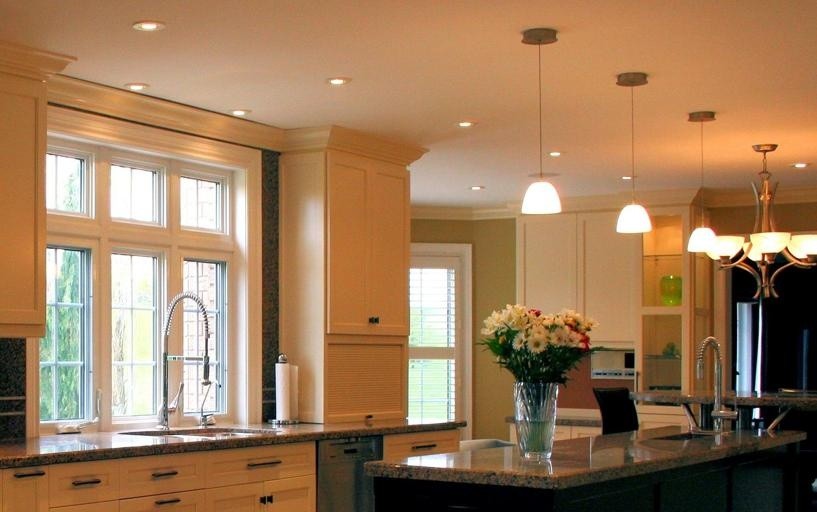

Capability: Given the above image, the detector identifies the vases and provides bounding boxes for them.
[512,382,558,458]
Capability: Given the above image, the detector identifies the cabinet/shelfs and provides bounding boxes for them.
[50,448,205,512]
[516,210,636,349]
[636,205,713,415]
[275,153,411,422]
[2,70,45,322]
[382,430,460,459]
[634,413,700,428]
[205,441,316,511]
[1,465,48,512]
[509,423,602,445]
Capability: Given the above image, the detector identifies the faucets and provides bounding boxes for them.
[696,336,740,434]
[159,291,212,427]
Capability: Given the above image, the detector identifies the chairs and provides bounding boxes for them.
[592,386,639,433]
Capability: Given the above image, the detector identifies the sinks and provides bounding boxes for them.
[648,431,711,441]
[118,428,282,437]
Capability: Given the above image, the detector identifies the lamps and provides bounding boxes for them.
[615,73,652,232]
[705,144,816,299]
[687,112,719,251]
[519,29,563,214]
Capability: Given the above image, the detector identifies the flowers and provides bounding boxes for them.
[474,305,606,422]
